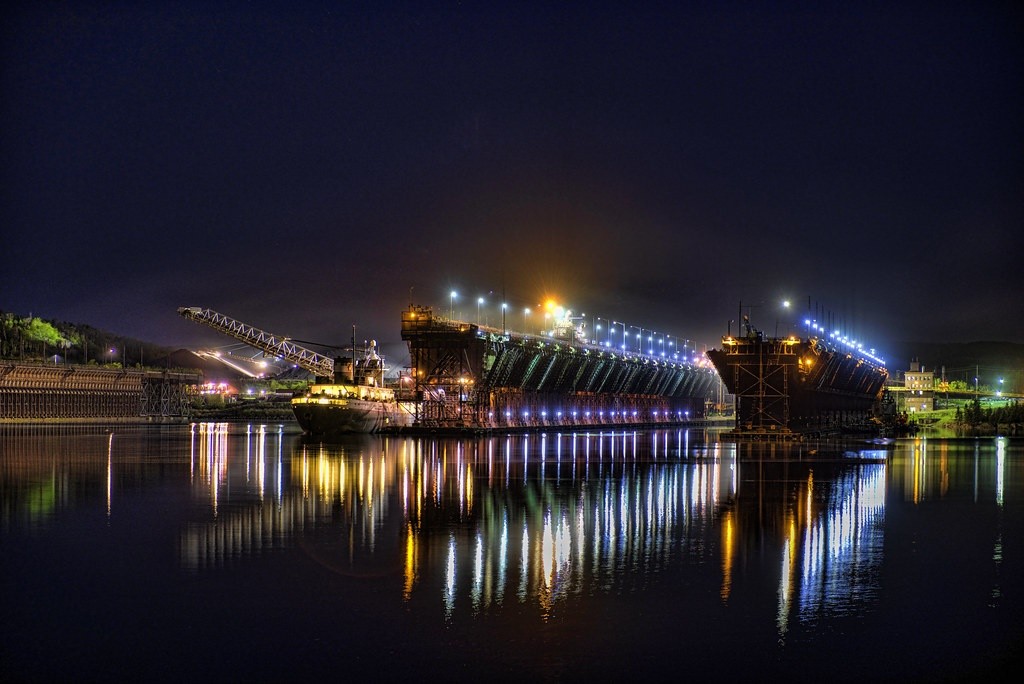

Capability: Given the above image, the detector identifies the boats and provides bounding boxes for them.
[175,300,417,439]
